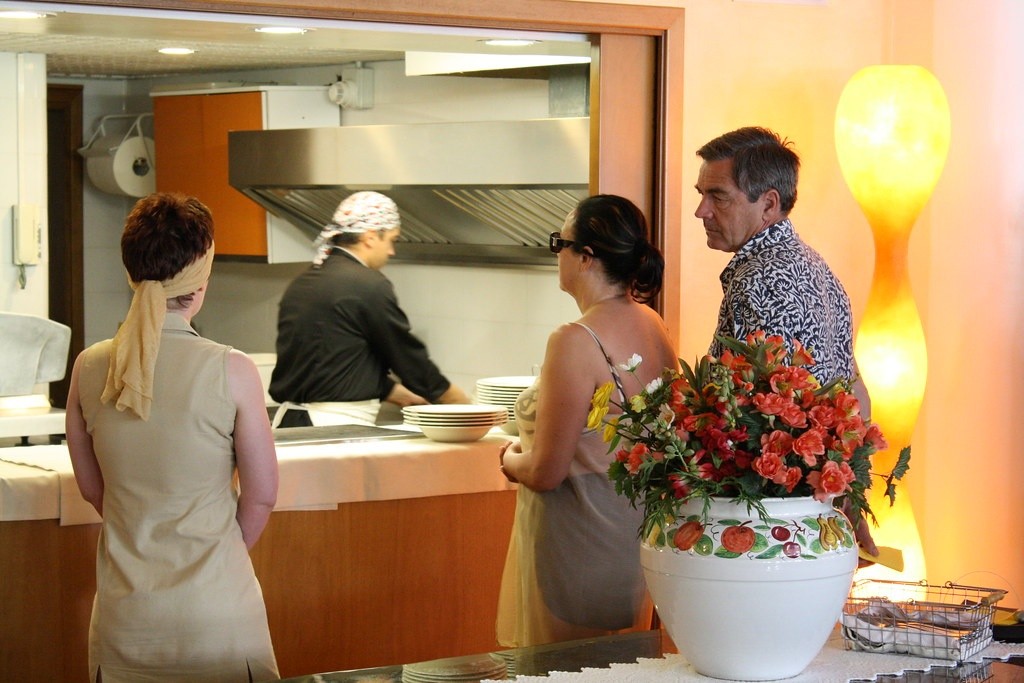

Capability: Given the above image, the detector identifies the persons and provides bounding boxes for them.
[495,195,681,647]
[694,129,878,568]
[267,190,470,431]
[66,193,282,683]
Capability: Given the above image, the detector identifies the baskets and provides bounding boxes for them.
[838,579,1009,662]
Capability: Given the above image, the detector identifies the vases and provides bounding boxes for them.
[639,492,858,680]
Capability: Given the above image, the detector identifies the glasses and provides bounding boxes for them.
[549,232,576,253]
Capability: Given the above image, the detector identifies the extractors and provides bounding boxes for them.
[226,118,591,265]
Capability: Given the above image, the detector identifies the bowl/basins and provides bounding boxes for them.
[402,649,516,683]
[476,376,545,436]
[400,403,510,442]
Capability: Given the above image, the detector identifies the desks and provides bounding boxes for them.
[265,630,1024,683]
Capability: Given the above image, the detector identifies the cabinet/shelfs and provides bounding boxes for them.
[148,84,341,265]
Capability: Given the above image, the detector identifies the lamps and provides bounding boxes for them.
[833,62,952,604]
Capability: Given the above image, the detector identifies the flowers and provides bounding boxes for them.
[582,329,913,533]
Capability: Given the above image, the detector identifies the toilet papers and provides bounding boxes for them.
[85,131,157,199]
